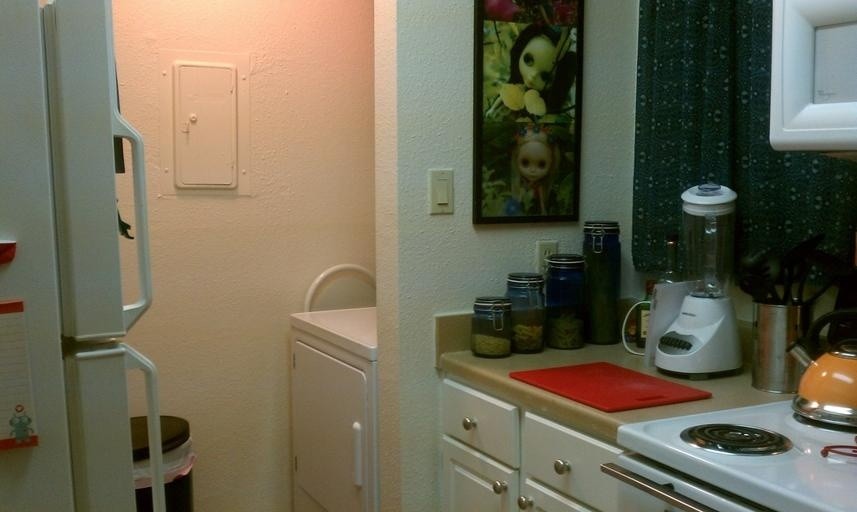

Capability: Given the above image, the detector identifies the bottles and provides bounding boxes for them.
[635,279,658,349]
[544,251,589,351]
[469,295,513,358]
[504,270,545,355]
[582,219,623,346]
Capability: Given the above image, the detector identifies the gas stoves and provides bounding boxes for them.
[616,400,856,511]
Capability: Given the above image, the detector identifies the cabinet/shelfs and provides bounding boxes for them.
[439,375,623,512]
[290,308,377,510]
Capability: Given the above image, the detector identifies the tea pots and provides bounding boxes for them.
[786,310,857,432]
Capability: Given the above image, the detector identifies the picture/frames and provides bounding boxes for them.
[472,1,580,224]
